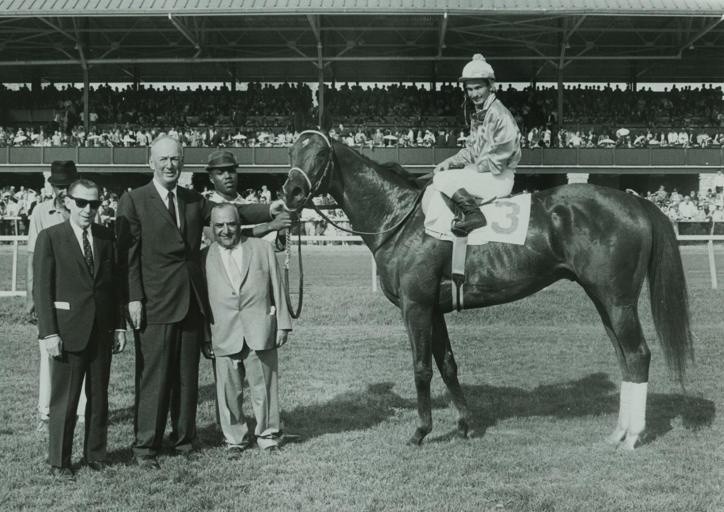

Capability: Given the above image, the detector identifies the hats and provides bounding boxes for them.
[206,150,239,171]
[47,160,81,186]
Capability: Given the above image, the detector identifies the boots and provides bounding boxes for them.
[451,188,486,237]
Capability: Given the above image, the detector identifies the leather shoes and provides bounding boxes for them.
[50,466,75,483]
[88,461,111,472]
[171,448,206,461]
[227,447,241,460]
[135,455,161,471]
[265,446,282,457]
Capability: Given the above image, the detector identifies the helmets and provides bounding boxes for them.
[459,54,496,81]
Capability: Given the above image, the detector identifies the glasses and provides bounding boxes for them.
[66,194,102,209]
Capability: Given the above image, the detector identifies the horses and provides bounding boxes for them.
[279,104,695,452]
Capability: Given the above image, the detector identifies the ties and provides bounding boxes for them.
[225,248,241,295]
[82,228,94,279]
[168,192,177,226]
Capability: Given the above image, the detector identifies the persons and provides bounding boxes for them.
[199,203,289,460]
[1,82,723,247]
[430,53,521,236]
[115,133,295,470]
[34,178,129,481]
[26,160,87,432]
[204,153,290,255]
